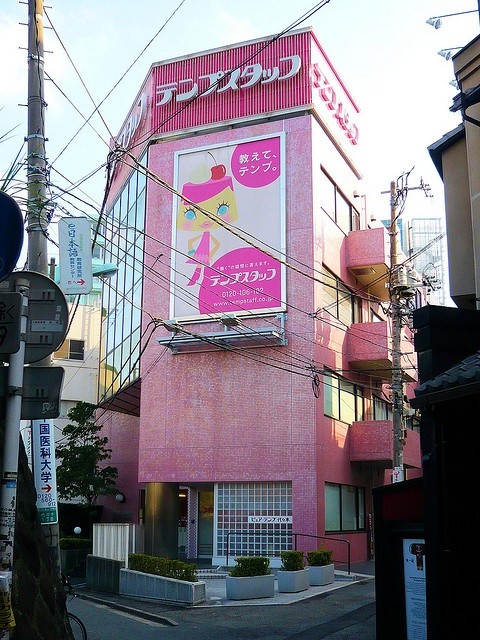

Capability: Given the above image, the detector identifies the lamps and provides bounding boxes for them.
[115,492,126,504]
